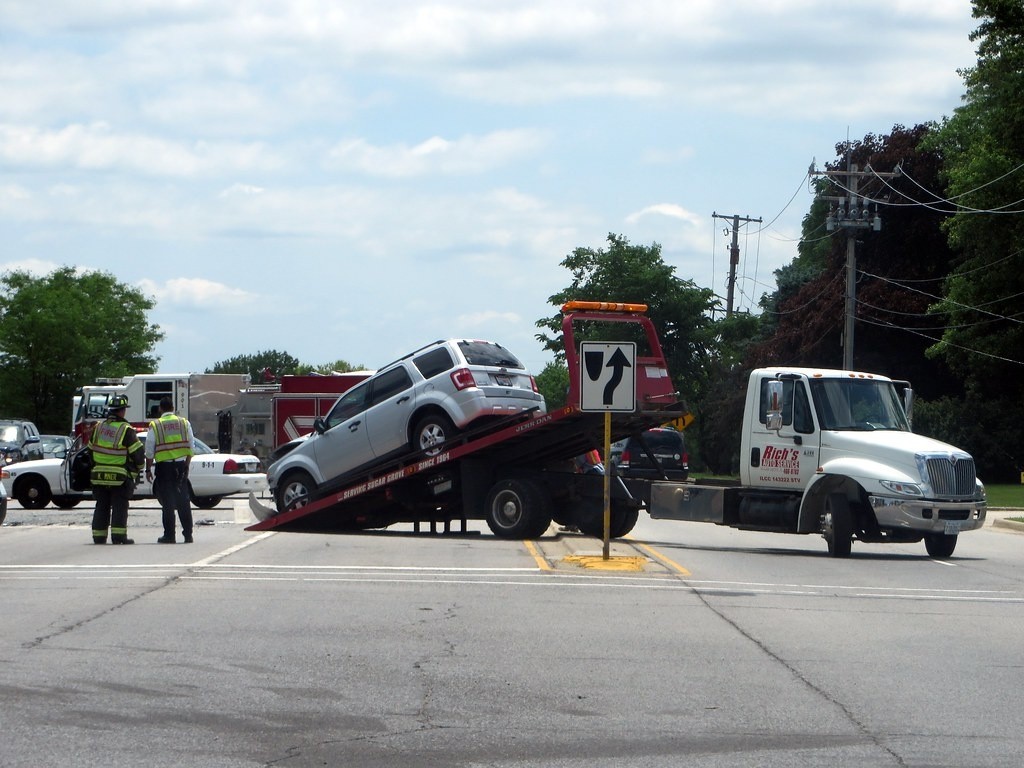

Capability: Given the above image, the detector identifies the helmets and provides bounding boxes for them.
[103,396,131,411]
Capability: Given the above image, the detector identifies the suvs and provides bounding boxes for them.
[265,339,546,516]
[0,419,45,466]
[609,426,689,481]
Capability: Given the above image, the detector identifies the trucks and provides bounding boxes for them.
[250,300,988,566]
[271,373,374,449]
[70,373,251,459]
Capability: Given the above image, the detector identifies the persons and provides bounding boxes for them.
[144,396,196,542]
[86,395,146,546]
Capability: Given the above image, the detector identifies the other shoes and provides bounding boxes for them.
[95,539,107,544]
[184,537,194,543]
[158,536,177,544]
[113,537,136,545]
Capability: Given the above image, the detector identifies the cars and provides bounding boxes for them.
[0,429,267,511]
[40,434,72,459]
